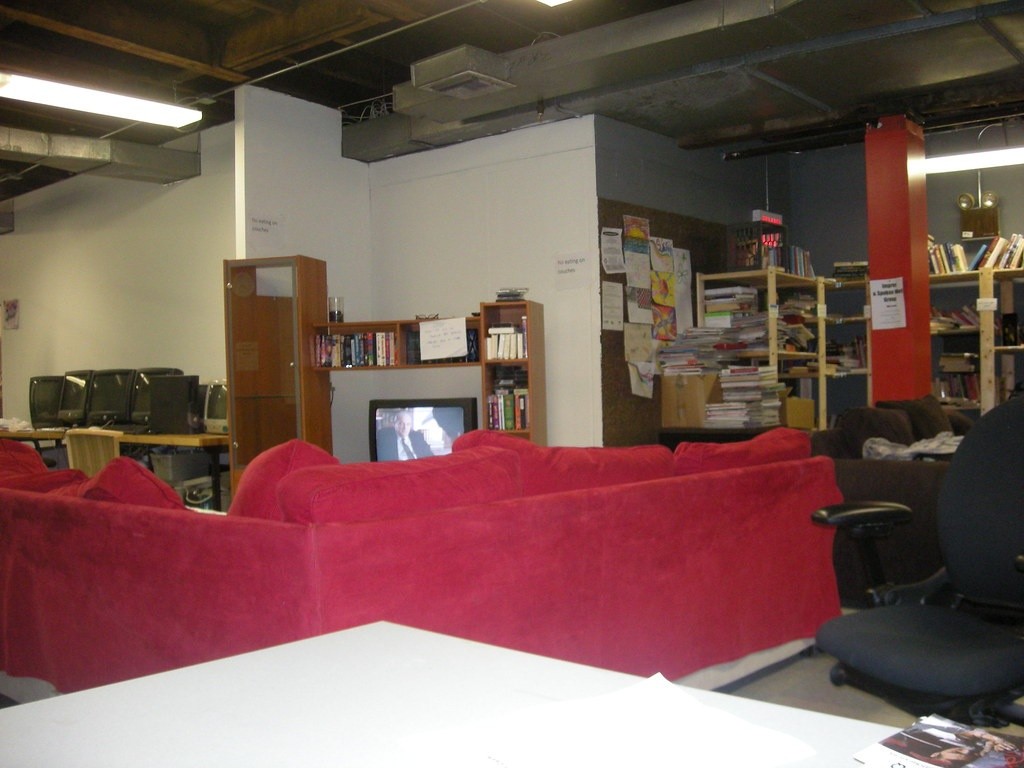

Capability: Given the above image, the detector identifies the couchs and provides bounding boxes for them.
[0,441,842,697]
[812,410,975,613]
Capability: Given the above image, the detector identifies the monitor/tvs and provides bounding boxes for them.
[29,368,228,435]
[369,397,477,463]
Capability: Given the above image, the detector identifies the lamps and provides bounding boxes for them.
[927,118,1024,174]
[958,189,1002,242]
[0,46,208,130]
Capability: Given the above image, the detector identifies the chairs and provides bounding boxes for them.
[65,428,125,478]
[809,393,1024,734]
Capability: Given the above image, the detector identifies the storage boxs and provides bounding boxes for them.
[152,451,212,483]
[703,311,734,328]
[660,373,723,428]
[780,386,817,428]
[833,260,869,280]
[736,221,790,274]
[939,354,976,372]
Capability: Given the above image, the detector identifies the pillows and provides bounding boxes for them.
[448,427,536,451]
[877,395,955,442]
[78,453,190,509]
[227,439,338,522]
[836,403,916,461]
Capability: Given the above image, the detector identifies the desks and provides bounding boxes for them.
[0,427,231,512]
[0,618,903,768]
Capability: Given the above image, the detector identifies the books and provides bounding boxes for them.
[310,332,398,368]
[484,316,527,360]
[400,328,479,365]
[853,712,1024,768]
[487,366,529,430]
[927,233,1024,400]
[657,246,868,429]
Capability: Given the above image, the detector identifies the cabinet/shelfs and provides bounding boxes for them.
[695,266,827,430]
[313,317,481,370]
[826,274,874,407]
[481,301,548,450]
[224,255,334,505]
[930,267,1024,418]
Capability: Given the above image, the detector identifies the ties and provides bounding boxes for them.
[401,438,415,459]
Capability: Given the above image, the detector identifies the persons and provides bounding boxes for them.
[376,407,463,460]
